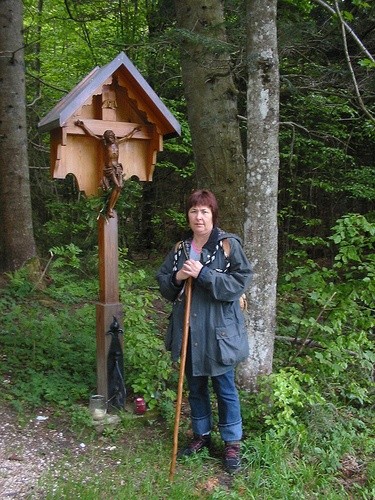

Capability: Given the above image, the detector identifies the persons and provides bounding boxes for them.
[74,118,143,216]
[155,190,254,472]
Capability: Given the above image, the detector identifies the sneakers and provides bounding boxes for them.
[224,442,242,474]
[178,434,212,458]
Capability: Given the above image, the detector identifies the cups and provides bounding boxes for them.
[89,395,106,421]
[135,397,146,415]
